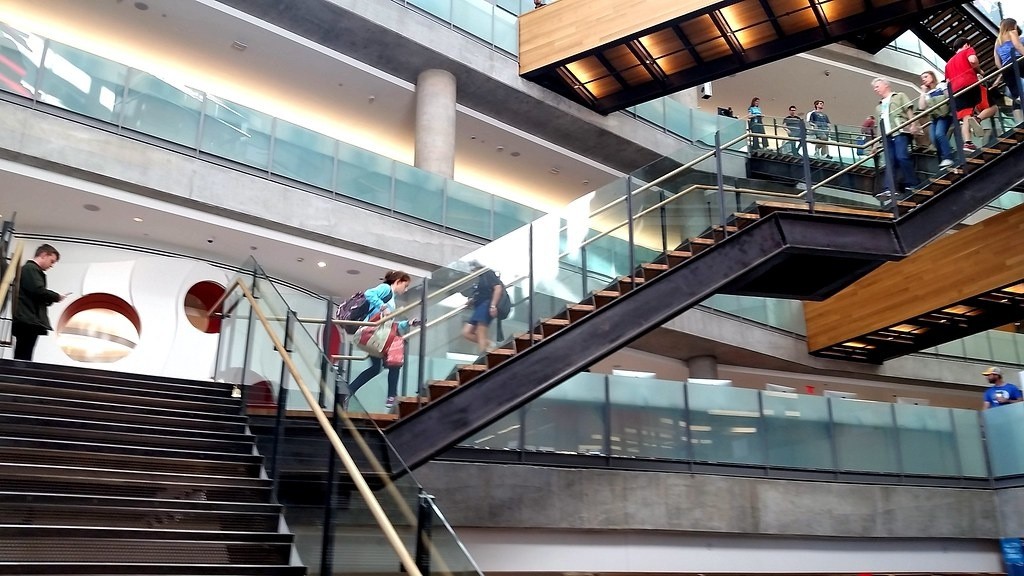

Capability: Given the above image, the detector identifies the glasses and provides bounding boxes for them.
[791,110,796,111]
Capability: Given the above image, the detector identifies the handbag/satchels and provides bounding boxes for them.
[336,290,392,334]
[354,310,405,367]
[496,280,511,320]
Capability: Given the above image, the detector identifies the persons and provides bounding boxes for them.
[918,71,955,171]
[723,107,739,119]
[806,100,833,159]
[460,258,502,365]
[11,244,67,360]
[861,115,881,158]
[945,37,998,149]
[871,77,925,197]
[748,96,773,151]
[981,366,1024,410]
[783,105,807,156]
[994,18,1024,123]
[341,270,417,408]
[534,0,547,9]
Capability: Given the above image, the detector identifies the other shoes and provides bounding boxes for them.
[386,397,394,408]
[939,159,953,170]
[962,143,977,153]
[968,115,985,137]
[876,190,899,200]
[826,155,832,159]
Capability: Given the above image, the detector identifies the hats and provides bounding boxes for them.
[982,367,1003,379]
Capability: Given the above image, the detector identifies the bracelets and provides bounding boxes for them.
[489,305,495,309]
